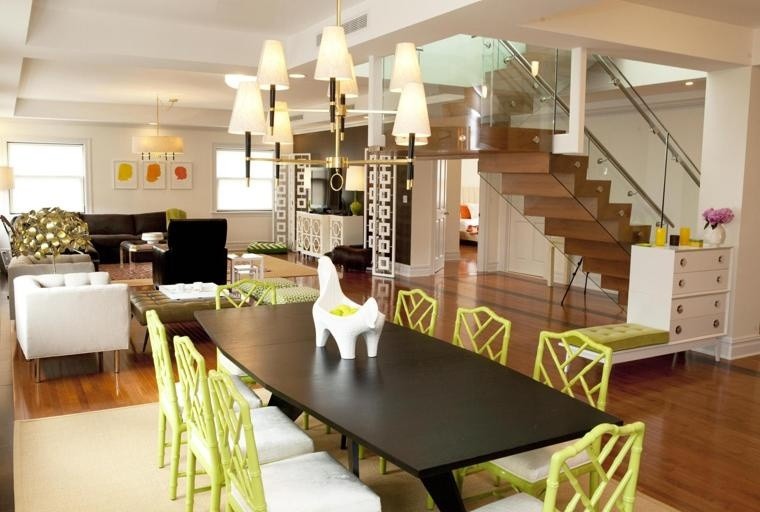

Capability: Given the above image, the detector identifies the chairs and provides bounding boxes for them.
[7,252,130,383]
[151,218,228,290]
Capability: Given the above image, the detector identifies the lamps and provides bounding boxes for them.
[227,1,433,193]
[344,165,365,216]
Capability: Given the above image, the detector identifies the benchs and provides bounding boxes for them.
[130,287,251,353]
[555,320,670,376]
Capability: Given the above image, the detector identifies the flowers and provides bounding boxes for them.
[701,208,735,229]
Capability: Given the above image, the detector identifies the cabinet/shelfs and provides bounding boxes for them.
[295,209,364,261]
[628,242,733,369]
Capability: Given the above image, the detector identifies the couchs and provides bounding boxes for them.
[82,210,168,264]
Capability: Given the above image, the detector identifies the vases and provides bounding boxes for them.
[708,223,726,245]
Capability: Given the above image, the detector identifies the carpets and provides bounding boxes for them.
[96,250,319,289]
[13,386,681,512]
